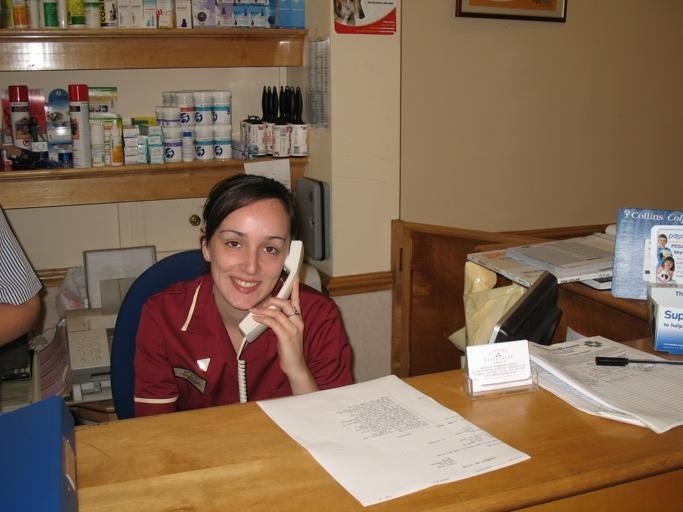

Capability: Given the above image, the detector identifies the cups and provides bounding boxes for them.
[240,119,308,159]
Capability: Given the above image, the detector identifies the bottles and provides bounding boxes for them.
[67,83,124,169]
[181,131,193,163]
[8,86,31,152]
[0,0,118,30]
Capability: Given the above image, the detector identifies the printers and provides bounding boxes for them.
[66,245,157,404]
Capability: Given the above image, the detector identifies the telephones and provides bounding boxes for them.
[239,241,305,343]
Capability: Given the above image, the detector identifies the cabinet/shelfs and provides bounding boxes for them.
[0,28,310,211]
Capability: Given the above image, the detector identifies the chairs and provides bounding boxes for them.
[111,249,288,420]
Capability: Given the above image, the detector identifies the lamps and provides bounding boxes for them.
[0,318,70,416]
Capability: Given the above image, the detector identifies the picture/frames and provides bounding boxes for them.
[454,0,569,24]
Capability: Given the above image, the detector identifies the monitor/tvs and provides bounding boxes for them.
[489,270,563,347]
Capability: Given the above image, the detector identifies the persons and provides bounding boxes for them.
[656,257,675,282]
[134,173,355,413]
[658,235,671,260]
[1,208,44,348]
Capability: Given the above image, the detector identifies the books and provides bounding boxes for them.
[467,248,613,287]
[507,232,616,278]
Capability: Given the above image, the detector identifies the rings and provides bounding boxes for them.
[289,309,300,318]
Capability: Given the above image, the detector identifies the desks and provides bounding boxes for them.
[75,243,683,512]
